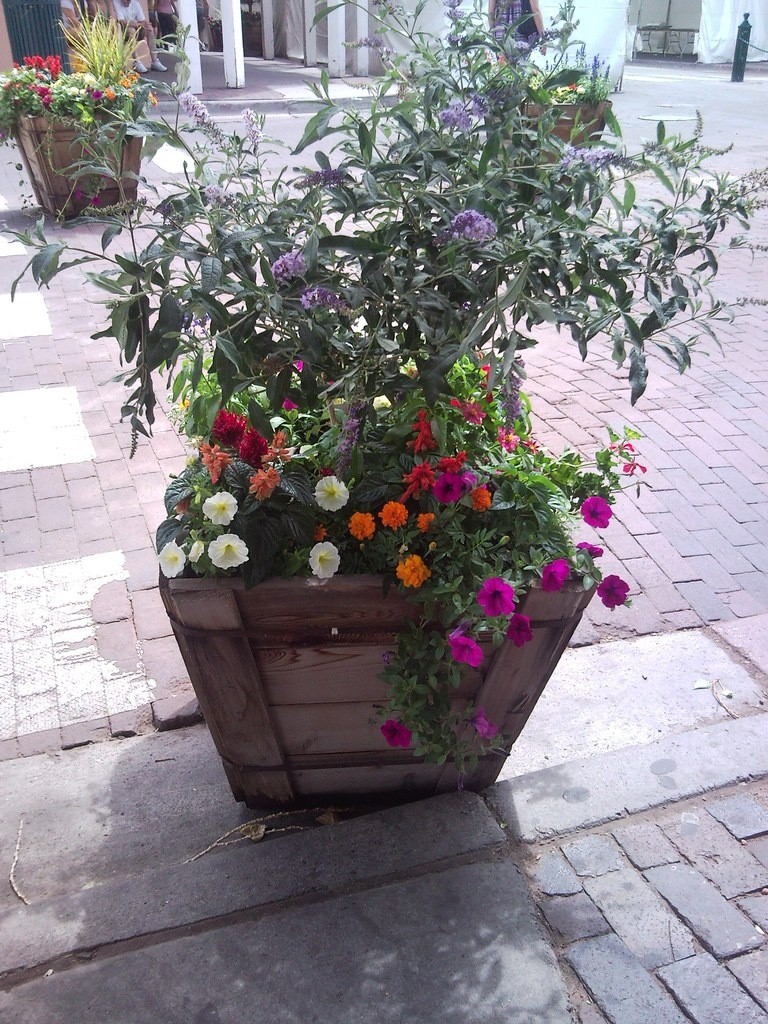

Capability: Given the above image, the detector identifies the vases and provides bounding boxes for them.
[15,114,143,218]
[522,100,613,187]
[159,485,597,809]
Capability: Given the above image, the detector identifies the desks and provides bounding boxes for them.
[637,27,699,60]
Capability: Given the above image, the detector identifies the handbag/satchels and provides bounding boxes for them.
[518,0,539,37]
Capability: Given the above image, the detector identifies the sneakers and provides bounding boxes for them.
[151,61,167,72]
[133,62,148,73]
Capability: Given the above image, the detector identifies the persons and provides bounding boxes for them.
[195,0,214,51]
[488,0,548,75]
[61,0,88,45]
[153,0,178,51]
[147,0,159,49]
[113,0,168,73]
[88,0,115,23]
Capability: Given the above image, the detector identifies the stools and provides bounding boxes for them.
[123,40,152,74]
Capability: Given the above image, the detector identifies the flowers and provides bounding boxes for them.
[483,45,609,106]
[0,0,768,783]
[0,0,157,206]
